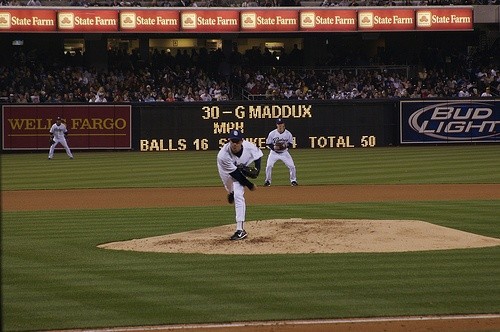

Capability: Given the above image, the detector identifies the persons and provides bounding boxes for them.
[0,0,41,6]
[264,119,297,186]
[217,130,263,240]
[0,48,500,104]
[320,0,500,7]
[68,0,271,8]
[48,117,74,159]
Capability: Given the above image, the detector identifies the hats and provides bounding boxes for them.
[276,118,284,124]
[228,130,242,141]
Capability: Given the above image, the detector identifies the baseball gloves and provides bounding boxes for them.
[274,142,288,151]
[238,163,259,179]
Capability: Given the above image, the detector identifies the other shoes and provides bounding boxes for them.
[290,181,297,187]
[263,181,270,186]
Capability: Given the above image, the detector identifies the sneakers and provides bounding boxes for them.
[229,230,248,240]
[227,191,234,204]
